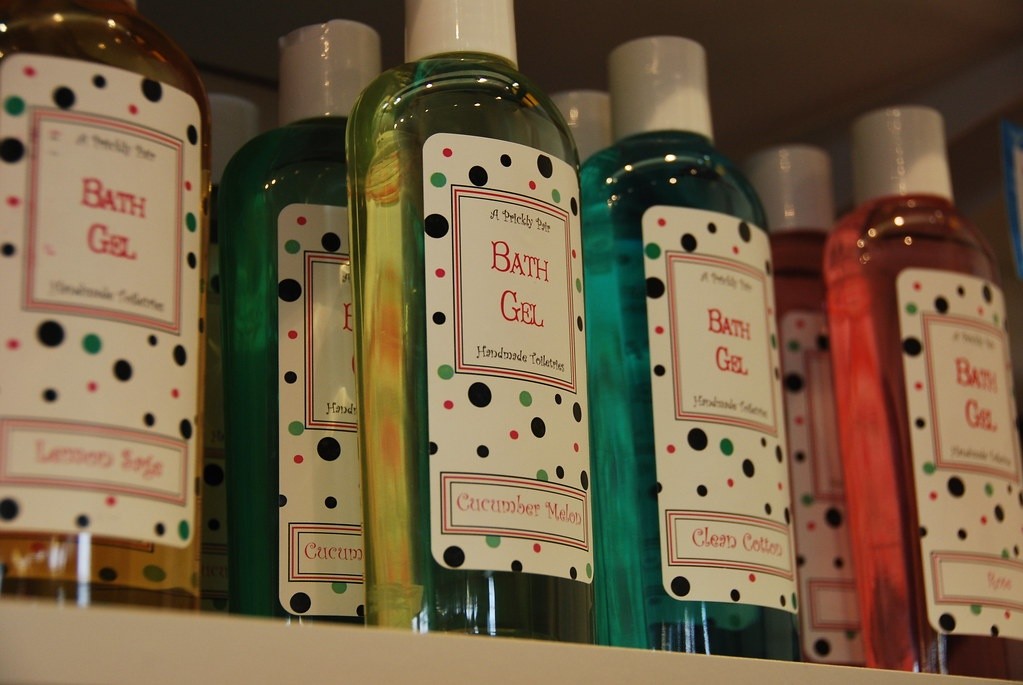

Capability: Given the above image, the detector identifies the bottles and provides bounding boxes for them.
[576,35,800,661]
[201,91,262,610]
[0,0,211,612]
[822,107,1023,684]
[551,88,613,166]
[345,0,596,647]
[215,19,382,626]
[739,146,859,666]
